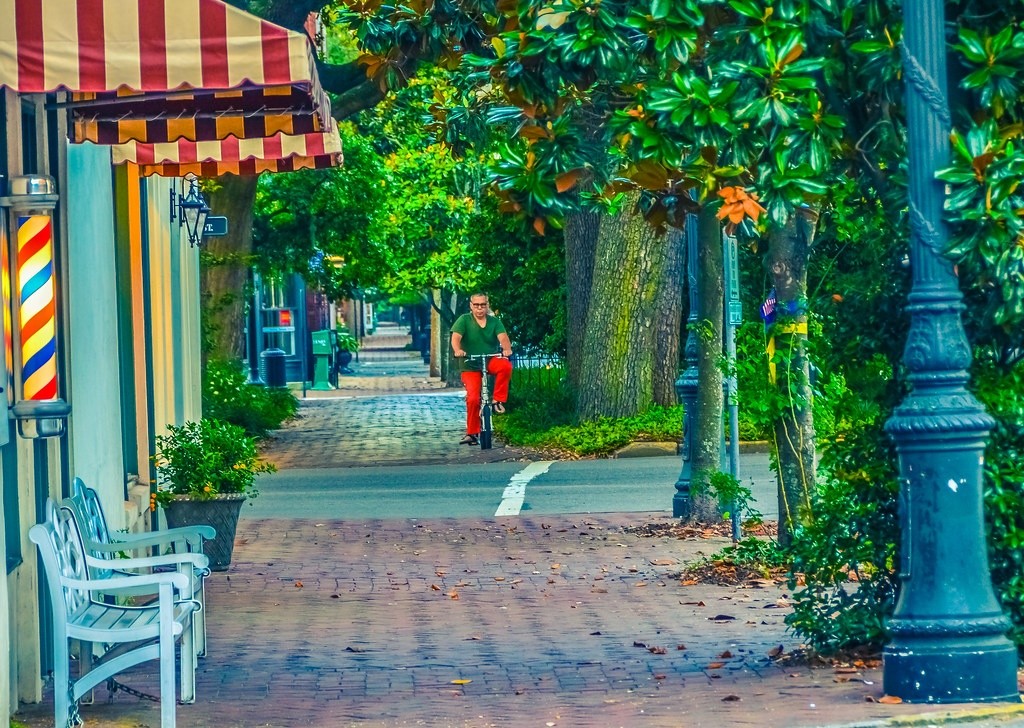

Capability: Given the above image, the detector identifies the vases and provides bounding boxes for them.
[160,492,248,573]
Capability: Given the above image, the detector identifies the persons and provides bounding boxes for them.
[450,291,513,445]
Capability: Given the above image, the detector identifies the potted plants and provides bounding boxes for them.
[335,323,358,374]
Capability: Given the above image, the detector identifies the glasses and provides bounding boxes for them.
[471,301,487,308]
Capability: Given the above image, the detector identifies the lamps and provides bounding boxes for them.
[169,178,212,249]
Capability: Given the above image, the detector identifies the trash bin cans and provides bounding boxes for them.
[260,348,288,390]
[310,329,338,390]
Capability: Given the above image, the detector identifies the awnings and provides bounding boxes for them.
[0,0,344,177]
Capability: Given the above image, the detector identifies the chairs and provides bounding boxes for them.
[28,476,217,728]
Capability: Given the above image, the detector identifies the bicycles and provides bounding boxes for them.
[453,346,509,449]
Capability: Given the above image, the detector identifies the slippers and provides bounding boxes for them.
[459,434,479,444]
[492,401,505,414]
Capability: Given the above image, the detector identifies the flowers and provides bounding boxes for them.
[150,417,277,513]
